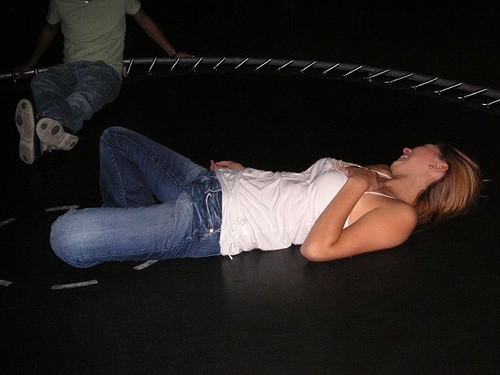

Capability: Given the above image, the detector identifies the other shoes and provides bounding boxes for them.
[15,99,41,164]
[35,117,80,152]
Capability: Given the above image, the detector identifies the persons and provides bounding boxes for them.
[13,1,200,168]
[48,123,478,274]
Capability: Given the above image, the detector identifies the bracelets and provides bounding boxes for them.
[168,51,178,59]
